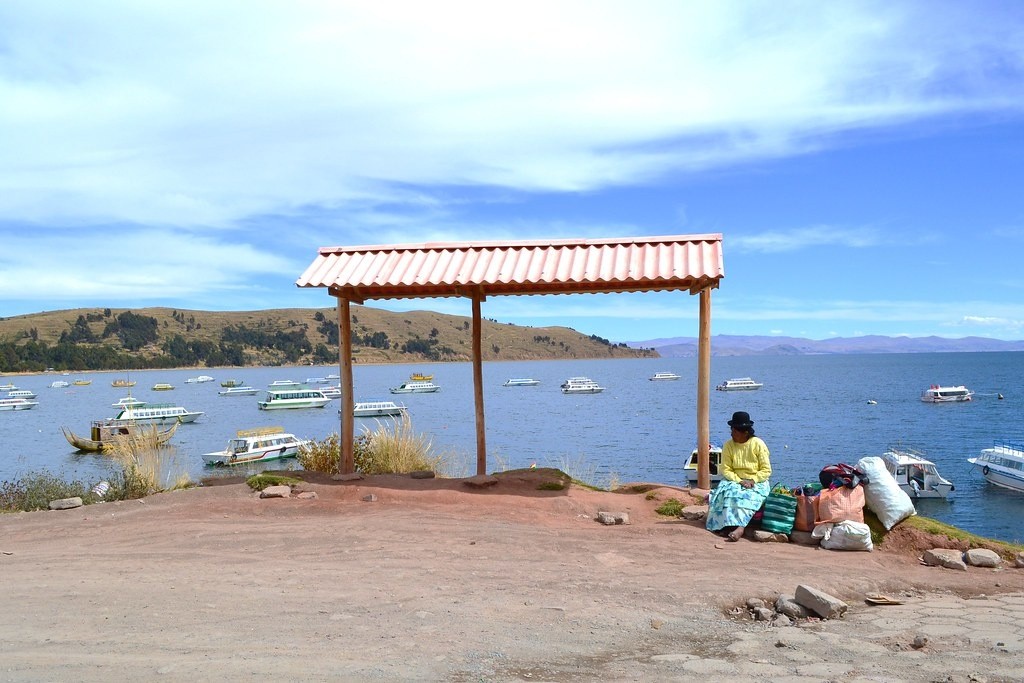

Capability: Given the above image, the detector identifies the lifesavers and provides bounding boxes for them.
[281,446,286,452]
[983,465,989,475]
[231,454,237,460]
[217,461,224,468]
[378,410,382,413]
[263,404,267,407]
[98,444,103,449]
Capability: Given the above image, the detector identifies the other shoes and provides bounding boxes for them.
[729,530,742,541]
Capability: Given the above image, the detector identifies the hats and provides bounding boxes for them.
[727,411,755,427]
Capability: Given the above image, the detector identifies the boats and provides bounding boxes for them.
[337,401,408,418]
[201,425,317,469]
[151,383,175,391]
[882,438,953,500]
[47,380,71,389]
[311,386,342,398]
[680,443,723,484]
[220,379,244,388]
[184,375,216,384]
[338,382,355,388]
[110,396,147,410]
[648,371,681,382]
[266,380,300,389]
[301,377,330,385]
[502,378,541,387]
[964,437,1024,494]
[0,396,40,411]
[217,385,262,396]
[559,376,607,394]
[919,384,976,402]
[409,374,434,381]
[325,375,340,379]
[409,372,434,381]
[715,376,764,391]
[8,390,38,399]
[111,380,136,388]
[388,380,441,394]
[71,374,93,386]
[0,382,21,392]
[255,385,332,411]
[105,401,205,426]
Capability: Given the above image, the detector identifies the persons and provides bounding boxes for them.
[706,412,773,542]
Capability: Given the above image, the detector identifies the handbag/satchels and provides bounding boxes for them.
[762,463,870,535]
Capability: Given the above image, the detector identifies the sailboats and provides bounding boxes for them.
[58,368,186,453]
